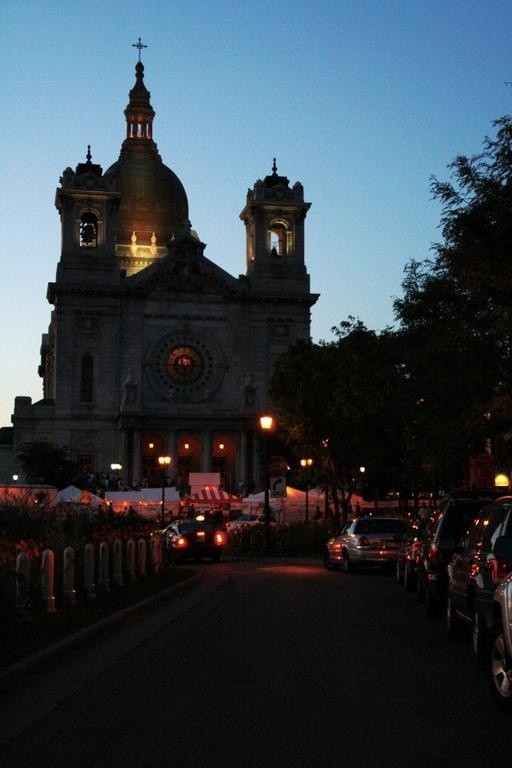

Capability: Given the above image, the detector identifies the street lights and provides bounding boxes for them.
[259,416,274,541]
[156,455,172,520]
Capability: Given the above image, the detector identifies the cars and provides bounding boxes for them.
[393,491,511,696]
[151,513,291,562]
[321,514,417,573]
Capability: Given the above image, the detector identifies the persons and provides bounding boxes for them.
[310,499,362,516]
[418,503,429,518]
